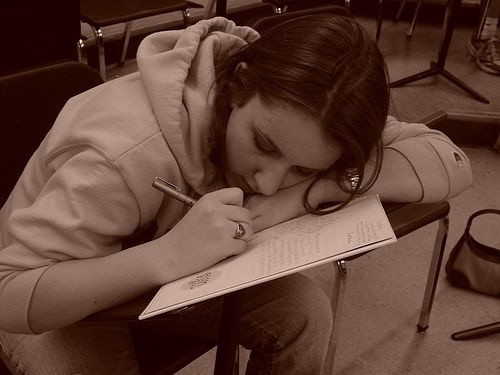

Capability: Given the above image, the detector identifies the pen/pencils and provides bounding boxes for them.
[152,177,258,238]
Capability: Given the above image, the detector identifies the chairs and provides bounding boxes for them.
[251,6,451,375]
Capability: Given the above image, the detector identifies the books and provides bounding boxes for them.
[138,193,397,320]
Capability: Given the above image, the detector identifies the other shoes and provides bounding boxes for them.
[465,30,500,74]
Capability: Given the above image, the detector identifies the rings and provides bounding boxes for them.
[234,220,246,240]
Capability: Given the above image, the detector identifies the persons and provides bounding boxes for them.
[466,0,500,75]
[0,12,473,375]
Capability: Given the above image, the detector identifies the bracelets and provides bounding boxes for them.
[344,166,363,200]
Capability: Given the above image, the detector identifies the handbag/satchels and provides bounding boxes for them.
[445,209,500,297]
